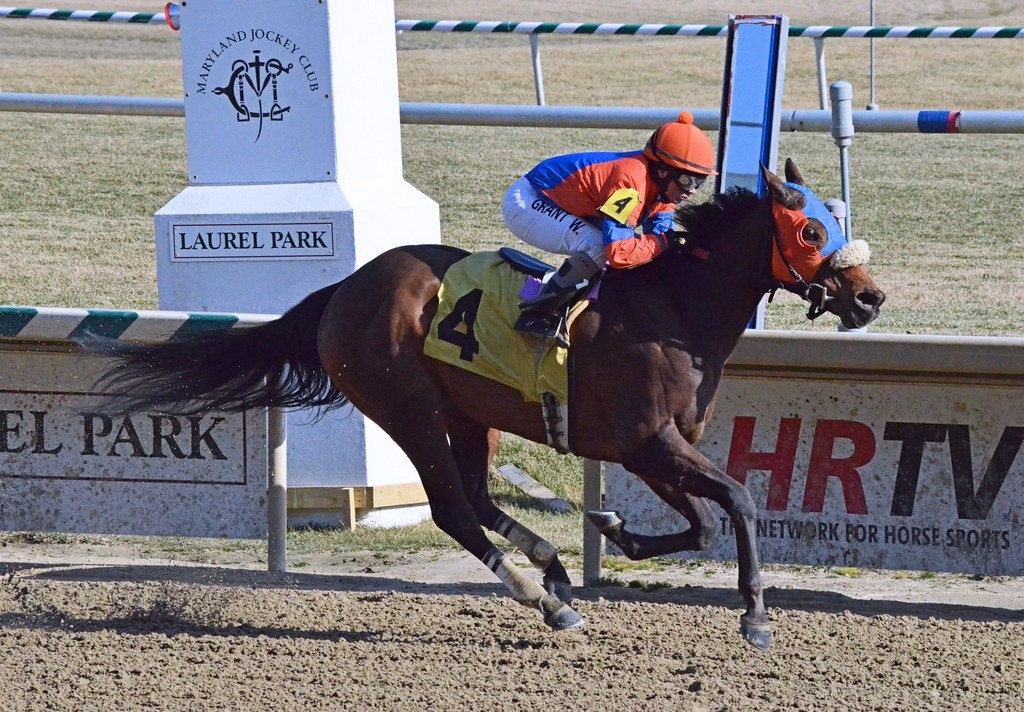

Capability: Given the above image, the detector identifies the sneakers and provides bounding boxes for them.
[515,308,570,348]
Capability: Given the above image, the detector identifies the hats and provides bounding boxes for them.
[643,112,719,175]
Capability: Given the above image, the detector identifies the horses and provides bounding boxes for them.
[66,156,888,654]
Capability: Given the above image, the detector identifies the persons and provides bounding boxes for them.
[502,111,719,340]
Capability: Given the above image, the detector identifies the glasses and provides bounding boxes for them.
[666,169,708,192]
